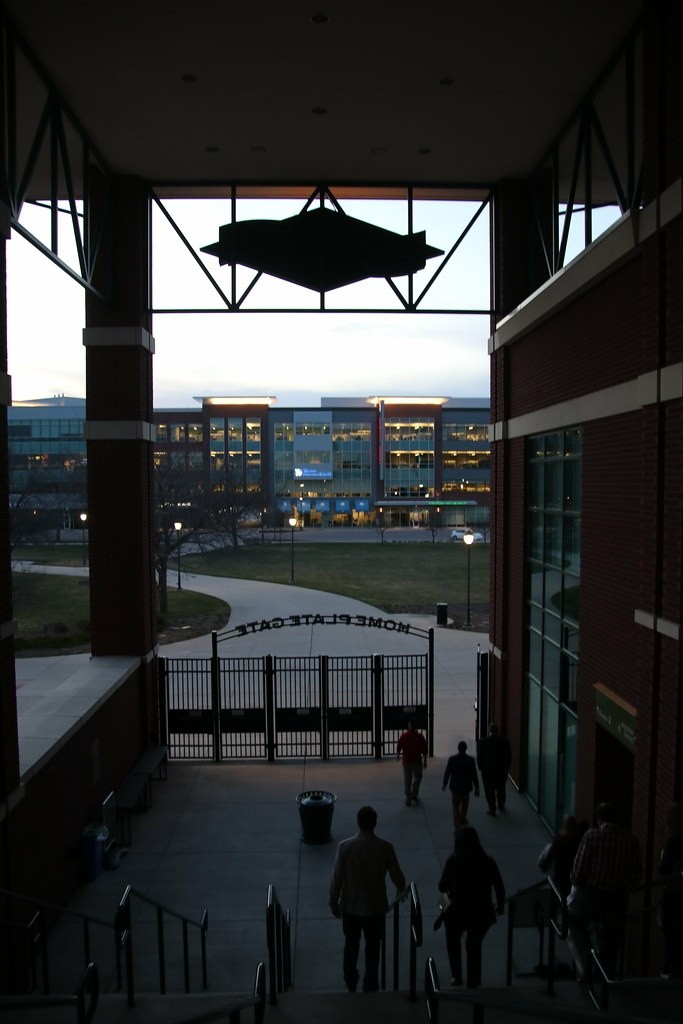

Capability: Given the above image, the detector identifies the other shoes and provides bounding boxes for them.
[463,819,468,825]
[450,978,463,987]
[405,799,411,806]
[466,984,479,990]
[660,972,673,982]
[411,793,417,800]
[498,806,505,812]
[346,981,359,992]
[363,984,379,992]
[486,809,496,817]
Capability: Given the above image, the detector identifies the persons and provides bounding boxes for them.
[396,717,428,808]
[441,740,480,836]
[328,806,407,994]
[478,721,514,818]
[657,802,683,980]
[438,826,506,991]
[536,799,643,985]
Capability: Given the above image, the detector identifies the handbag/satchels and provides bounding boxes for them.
[656,903,665,929]
[537,843,552,873]
[433,911,445,931]
[566,828,597,913]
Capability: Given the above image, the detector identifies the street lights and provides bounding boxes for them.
[288,517,297,583]
[173,521,183,593]
[463,529,475,627]
[80,513,88,567]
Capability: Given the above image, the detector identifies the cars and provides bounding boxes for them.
[452,529,483,540]
